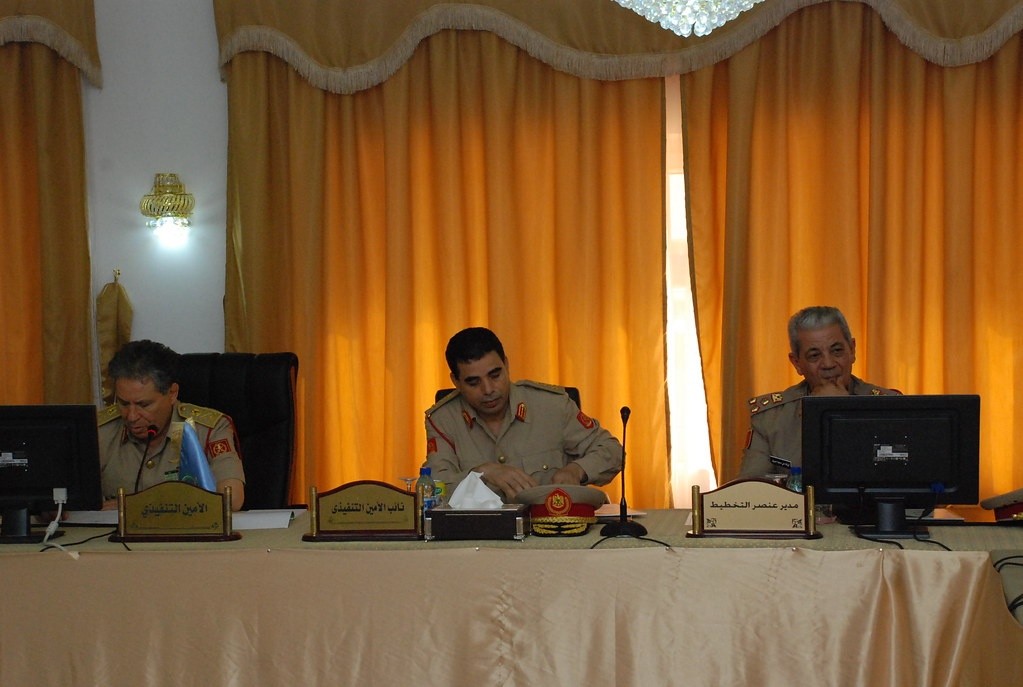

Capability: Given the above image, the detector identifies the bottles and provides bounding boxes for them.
[415,466,435,535]
[785,467,803,494]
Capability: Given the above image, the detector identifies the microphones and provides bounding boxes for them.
[600,407,648,537]
[135,425,159,493]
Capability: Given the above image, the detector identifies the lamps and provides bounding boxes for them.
[139,172,195,251]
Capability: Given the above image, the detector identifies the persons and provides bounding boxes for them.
[93,341,245,513]
[741,305,902,485]
[416,324,629,499]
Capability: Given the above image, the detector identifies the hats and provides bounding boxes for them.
[981,487,1023,521]
[517,482,608,539]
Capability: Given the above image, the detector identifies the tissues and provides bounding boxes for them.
[424,469,535,544]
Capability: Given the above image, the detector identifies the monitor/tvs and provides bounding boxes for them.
[0,405,104,511]
[801,394,981,505]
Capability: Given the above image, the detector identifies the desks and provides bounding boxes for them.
[0,510,1023,687]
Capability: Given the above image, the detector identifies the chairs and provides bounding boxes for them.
[175,351,298,513]
[435,384,581,413]
